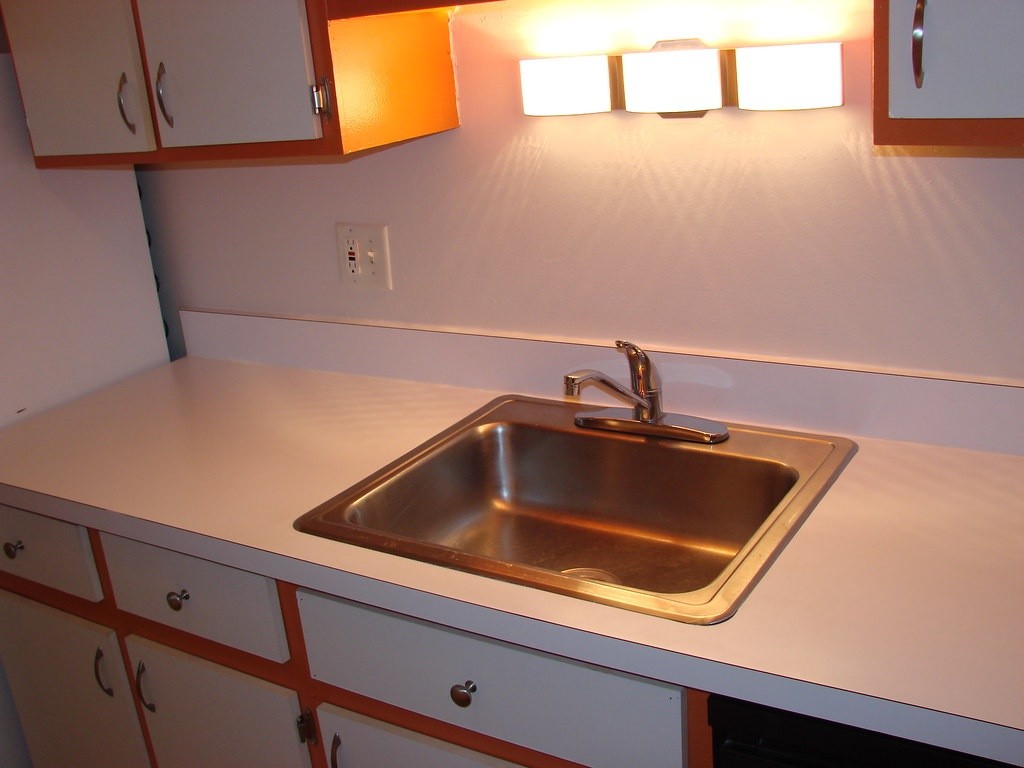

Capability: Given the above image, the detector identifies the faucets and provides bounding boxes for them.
[563,339,730,444]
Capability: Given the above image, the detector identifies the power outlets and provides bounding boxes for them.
[334,221,394,292]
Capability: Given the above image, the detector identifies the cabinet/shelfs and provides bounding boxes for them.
[0,482,312,767]
[0,0,344,168]
[872,0,1023,145]
[294,559,690,768]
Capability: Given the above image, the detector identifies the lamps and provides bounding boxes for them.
[519,36,845,118]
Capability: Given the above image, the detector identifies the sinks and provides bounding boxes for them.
[293,393,860,627]
